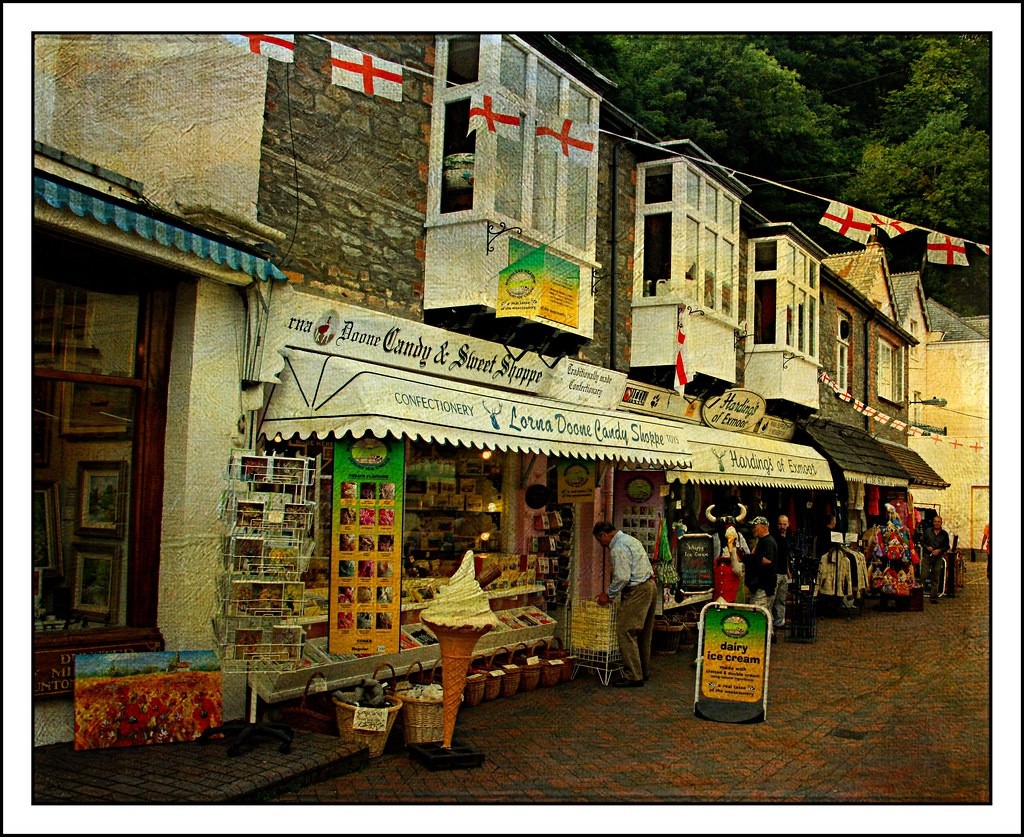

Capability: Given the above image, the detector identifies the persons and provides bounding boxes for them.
[920,517,950,603]
[980,523,990,585]
[734,515,792,644]
[592,520,658,688]
[816,515,836,559]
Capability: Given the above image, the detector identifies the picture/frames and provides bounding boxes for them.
[60,337,138,442]
[32,480,65,579]
[65,541,123,624]
[33,377,54,467]
[73,461,129,542]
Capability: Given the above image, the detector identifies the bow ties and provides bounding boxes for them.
[716,557,732,566]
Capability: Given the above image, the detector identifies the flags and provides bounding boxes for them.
[818,372,984,454]
[819,201,990,266]
[222,33,598,169]
[674,319,696,398]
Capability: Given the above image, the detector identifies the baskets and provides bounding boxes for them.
[529,640,561,686]
[671,611,698,649]
[261,671,329,736]
[332,663,402,759]
[509,643,539,691]
[394,661,445,744]
[463,670,486,708]
[470,655,502,701]
[490,647,522,697]
[548,636,572,682]
[653,616,683,653]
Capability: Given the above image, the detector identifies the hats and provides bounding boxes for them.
[749,516,769,525]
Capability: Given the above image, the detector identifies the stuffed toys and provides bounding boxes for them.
[865,502,920,596]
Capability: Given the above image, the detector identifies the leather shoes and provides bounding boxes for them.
[615,678,643,687]
[642,675,649,681]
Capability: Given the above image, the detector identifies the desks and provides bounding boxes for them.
[664,592,713,610]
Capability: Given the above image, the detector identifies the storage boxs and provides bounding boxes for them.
[303,458,537,617]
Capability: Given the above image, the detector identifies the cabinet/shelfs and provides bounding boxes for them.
[403,473,501,560]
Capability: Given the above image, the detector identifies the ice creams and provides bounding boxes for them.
[419,547,501,749]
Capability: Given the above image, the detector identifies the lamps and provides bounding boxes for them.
[910,396,948,407]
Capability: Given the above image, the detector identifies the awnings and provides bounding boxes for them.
[255,347,693,471]
[666,425,834,490]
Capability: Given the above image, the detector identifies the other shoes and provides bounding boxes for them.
[776,624,789,629]
[771,635,778,644]
[930,597,938,604]
